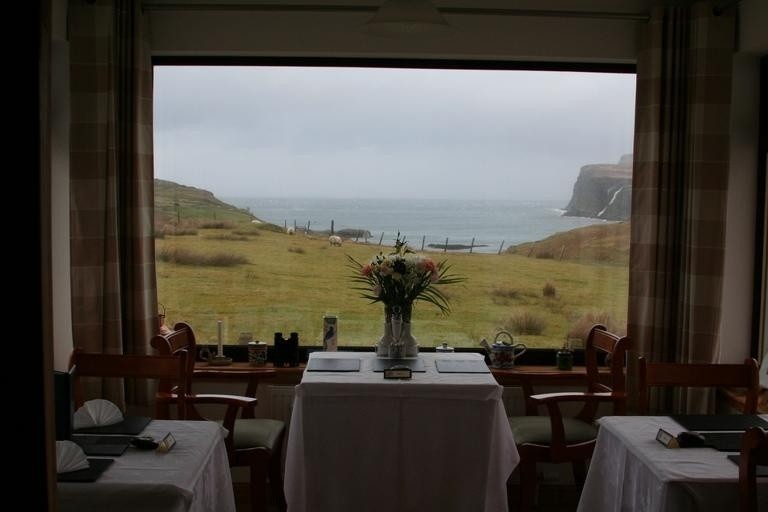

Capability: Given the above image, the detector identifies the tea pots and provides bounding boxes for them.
[480,331,527,369]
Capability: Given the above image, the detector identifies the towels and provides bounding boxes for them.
[72,398,125,430]
[56,440,90,475]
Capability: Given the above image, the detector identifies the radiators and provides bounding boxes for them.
[265,385,526,475]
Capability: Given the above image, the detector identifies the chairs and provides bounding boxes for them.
[150,322,287,512]
[69,345,189,420]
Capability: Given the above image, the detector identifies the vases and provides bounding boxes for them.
[376,318,418,358]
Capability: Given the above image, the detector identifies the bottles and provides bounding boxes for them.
[556,345,574,369]
[247,340,268,368]
[435,343,455,353]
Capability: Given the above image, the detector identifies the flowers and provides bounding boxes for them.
[343,227,471,322]
